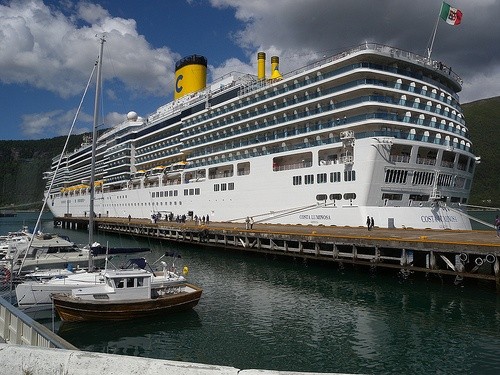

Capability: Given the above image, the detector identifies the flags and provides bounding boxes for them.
[438,2,463,26]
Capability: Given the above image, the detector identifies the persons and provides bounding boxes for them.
[245,216,251,230]
[84,209,212,226]
[494,215,500,239]
[370,217,374,229]
[366,216,370,231]
[250,217,254,229]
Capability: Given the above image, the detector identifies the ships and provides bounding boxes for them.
[43,40,500,280]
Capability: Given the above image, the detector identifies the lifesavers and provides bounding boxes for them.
[0,268,11,283]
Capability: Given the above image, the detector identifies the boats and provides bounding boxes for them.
[50,269,203,326]
[0,207,17,217]
[19,266,185,319]
[0,225,111,294]
